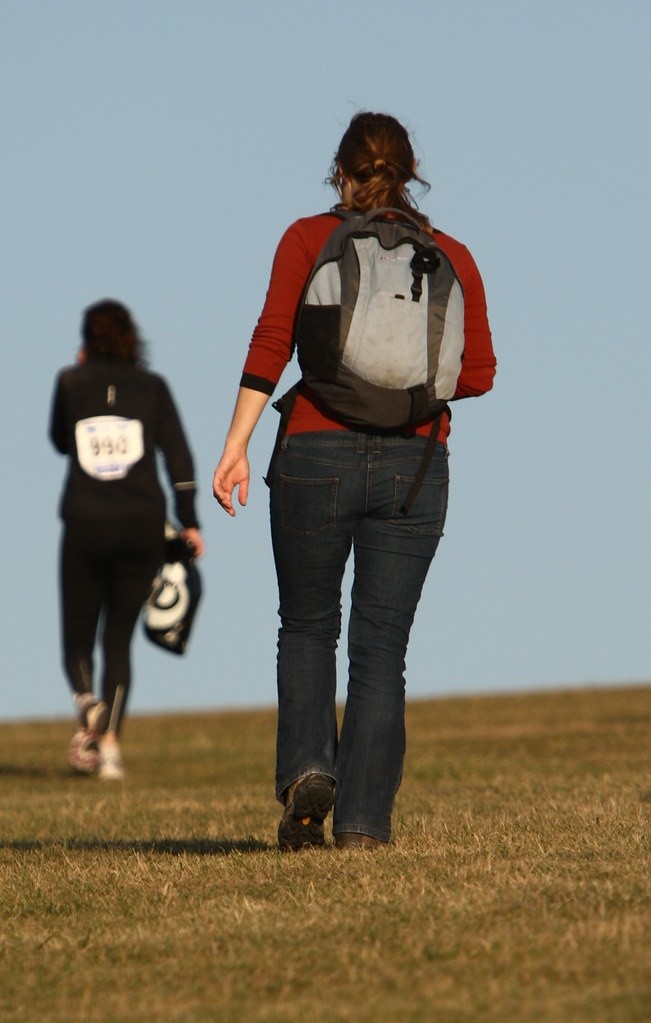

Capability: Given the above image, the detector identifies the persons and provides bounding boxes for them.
[48,297,201,774]
[214,112,497,840]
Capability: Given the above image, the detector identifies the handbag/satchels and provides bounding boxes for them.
[139,534,200,657]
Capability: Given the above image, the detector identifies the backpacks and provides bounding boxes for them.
[294,203,465,437]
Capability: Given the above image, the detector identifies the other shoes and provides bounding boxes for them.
[96,738,123,781]
[276,764,338,854]
[332,830,383,853]
[65,697,107,778]
